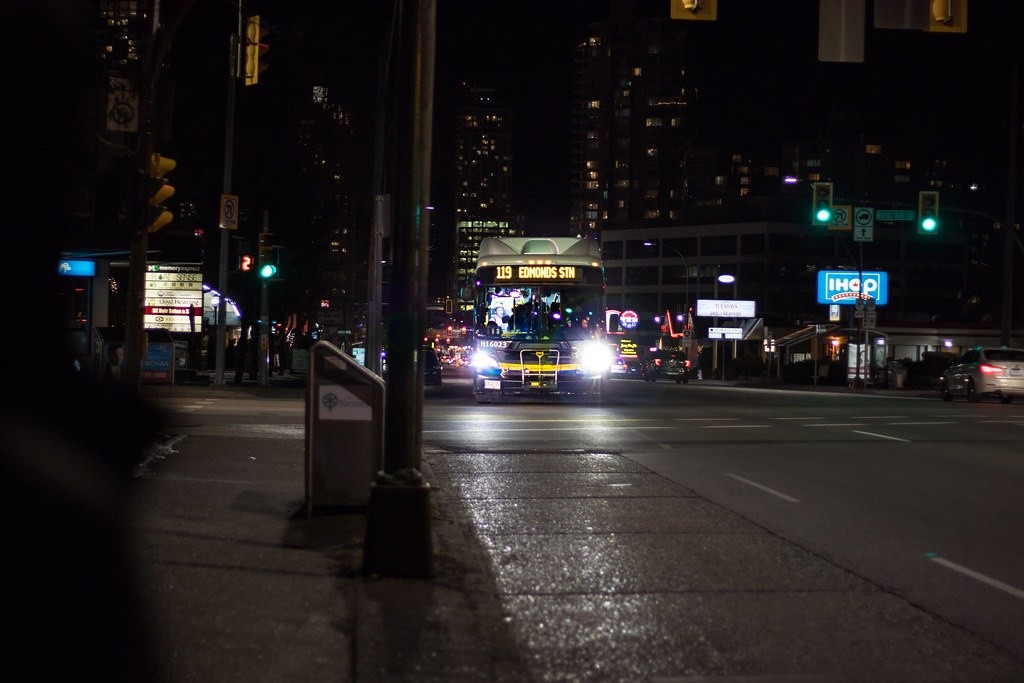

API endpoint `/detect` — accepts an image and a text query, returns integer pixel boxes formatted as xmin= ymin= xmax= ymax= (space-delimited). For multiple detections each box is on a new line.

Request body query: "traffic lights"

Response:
xmin=245 ymin=15 xmax=275 ymax=86
xmin=143 ymin=151 xmax=180 ymax=235
xmin=811 ymin=181 xmax=833 ymax=226
xmin=447 ymin=326 xmax=454 ymax=337
xmin=445 ymin=299 xmax=453 ymax=314
xmin=462 ymin=326 xmax=467 ymax=334
xmin=258 ymin=233 xmax=280 ymax=280
xmin=239 ymin=255 xmax=257 ymax=272
xmin=918 ymin=190 xmax=941 ymax=242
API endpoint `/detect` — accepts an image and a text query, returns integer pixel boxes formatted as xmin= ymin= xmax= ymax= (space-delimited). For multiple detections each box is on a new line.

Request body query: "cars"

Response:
xmin=938 ymin=347 xmax=1024 ymax=404
xmin=422 ymin=348 xmax=445 ymax=385
xmin=643 ymin=350 xmax=693 ymax=383
xmin=440 ymin=353 xmax=472 ymax=365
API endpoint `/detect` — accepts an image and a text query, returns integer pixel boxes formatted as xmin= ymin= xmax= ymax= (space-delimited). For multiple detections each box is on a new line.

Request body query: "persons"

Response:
xmin=106 ymin=342 xmax=176 ymax=444
xmin=509 ymin=305 xmax=527 ymax=332
xmin=491 ymin=306 xmax=504 ymax=326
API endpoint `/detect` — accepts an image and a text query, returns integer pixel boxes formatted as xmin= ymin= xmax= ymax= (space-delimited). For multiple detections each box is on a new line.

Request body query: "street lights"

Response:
xmin=644 ymin=241 xmax=690 ymax=360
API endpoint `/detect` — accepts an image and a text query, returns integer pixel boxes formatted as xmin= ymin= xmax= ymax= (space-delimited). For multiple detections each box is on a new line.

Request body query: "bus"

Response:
xmin=473 ymin=239 xmax=613 ymax=405
